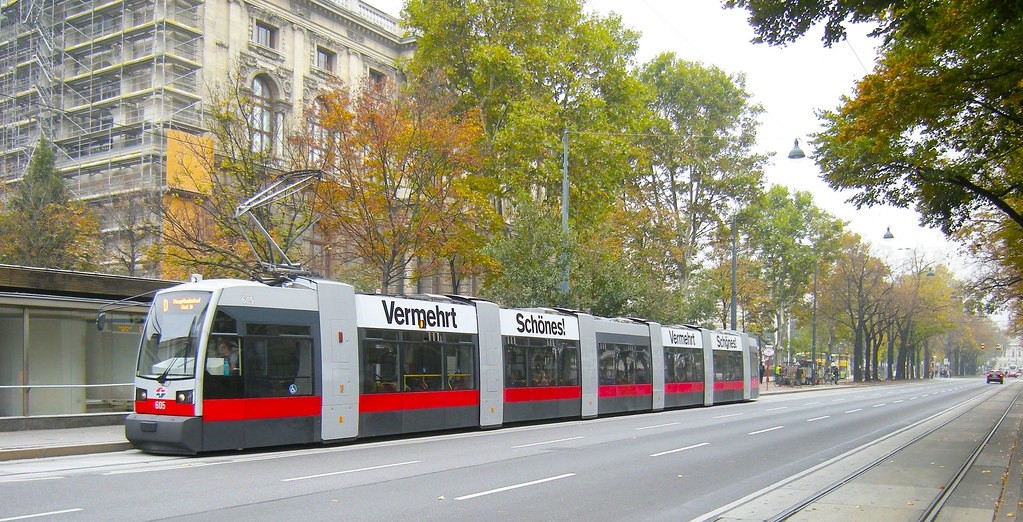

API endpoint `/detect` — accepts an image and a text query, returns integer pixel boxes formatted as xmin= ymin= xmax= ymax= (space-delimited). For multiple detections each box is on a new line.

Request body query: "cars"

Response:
xmin=986 ymin=369 xmax=1004 ymax=384
xmin=1002 ymin=364 xmax=1023 ymax=378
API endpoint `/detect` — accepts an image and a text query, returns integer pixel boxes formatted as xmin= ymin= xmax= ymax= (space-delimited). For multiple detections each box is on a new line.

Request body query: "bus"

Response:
xmin=94 ymin=169 xmax=760 ymax=457
xmin=793 ymin=350 xmax=849 ymax=380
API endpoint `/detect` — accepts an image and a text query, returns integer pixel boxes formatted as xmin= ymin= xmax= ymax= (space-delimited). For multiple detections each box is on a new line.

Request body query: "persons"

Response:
xmin=757 ymin=362 xmax=838 ymax=385
xmin=447 ymin=366 xmax=619 ymax=390
xmin=928 ymin=366 xmax=952 ymax=378
xmin=219 ymin=344 xmax=240 ymax=373
